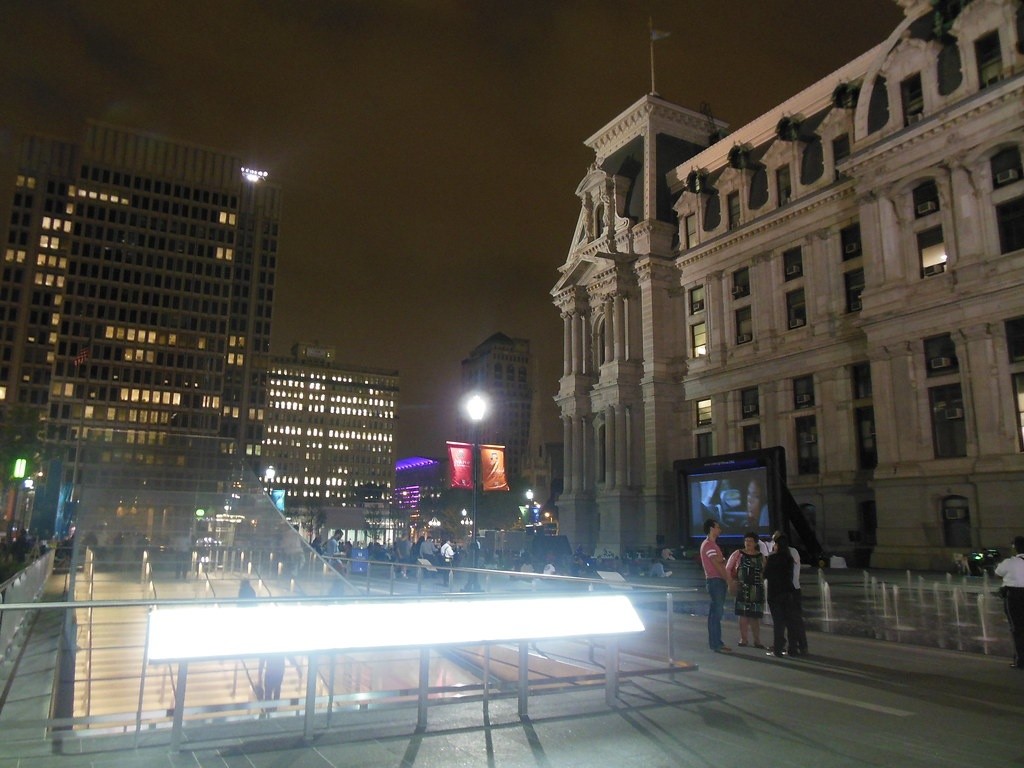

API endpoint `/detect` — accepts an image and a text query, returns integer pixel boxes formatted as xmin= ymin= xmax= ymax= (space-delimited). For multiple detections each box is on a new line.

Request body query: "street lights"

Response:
xmin=4 ymin=458 xmax=27 ymax=562
xmin=253 ymin=465 xmax=279 ymax=575
xmin=454 ymin=386 xmax=495 ymax=594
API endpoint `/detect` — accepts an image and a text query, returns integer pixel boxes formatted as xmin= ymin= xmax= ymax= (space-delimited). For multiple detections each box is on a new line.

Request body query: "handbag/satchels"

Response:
xmin=443 ymin=556 xmax=453 ymax=564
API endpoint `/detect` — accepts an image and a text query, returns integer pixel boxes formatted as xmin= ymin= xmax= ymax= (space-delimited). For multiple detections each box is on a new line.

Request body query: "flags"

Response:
xmin=480 ymin=449 xmax=510 ymax=491
xmin=448 ymin=447 xmax=474 ymax=491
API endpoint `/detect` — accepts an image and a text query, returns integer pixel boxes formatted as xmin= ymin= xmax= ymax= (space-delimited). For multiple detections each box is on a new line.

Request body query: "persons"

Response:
xmin=739 ymin=472 xmax=765 ymax=529
xmin=237 ymin=581 xmax=256 ymax=608
xmin=700 ymin=519 xmax=808 ymax=657
xmin=275 ymin=525 xmax=687 ymax=587
xmin=64 ymin=530 xmax=191 ymax=580
xmin=255 ymin=656 xmax=285 ymax=711
xmin=995 ymin=536 xmax=1024 ymax=668
xmin=0 ymin=530 xmax=46 ymax=575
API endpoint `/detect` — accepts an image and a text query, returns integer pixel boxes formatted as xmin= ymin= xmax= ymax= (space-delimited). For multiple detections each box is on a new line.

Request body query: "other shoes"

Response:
xmin=752 ymin=642 xmax=766 ymax=649
xmin=714 ymin=644 xmax=733 ymax=653
xmin=401 ymin=570 xmax=406 ymax=578
xmin=737 ymin=639 xmax=748 ymax=646
xmin=766 ymin=649 xmax=781 ymax=657
xmin=1010 ymin=663 xmax=1024 ymax=668
